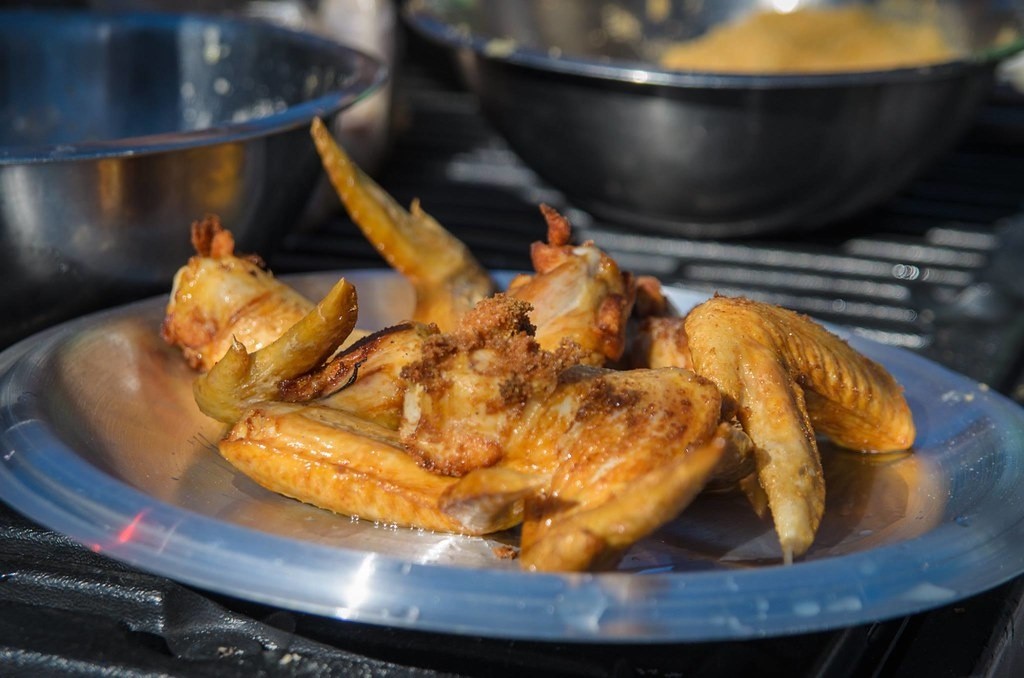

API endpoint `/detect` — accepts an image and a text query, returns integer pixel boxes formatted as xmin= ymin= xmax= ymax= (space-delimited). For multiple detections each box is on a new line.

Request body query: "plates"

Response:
xmin=3 ymin=267 xmax=1024 ymax=648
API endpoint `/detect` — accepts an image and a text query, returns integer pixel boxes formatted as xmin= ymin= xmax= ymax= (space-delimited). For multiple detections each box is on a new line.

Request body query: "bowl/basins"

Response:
xmin=395 ymin=4 xmax=1016 ymax=237
xmin=1 ymin=14 xmax=391 ymax=312
